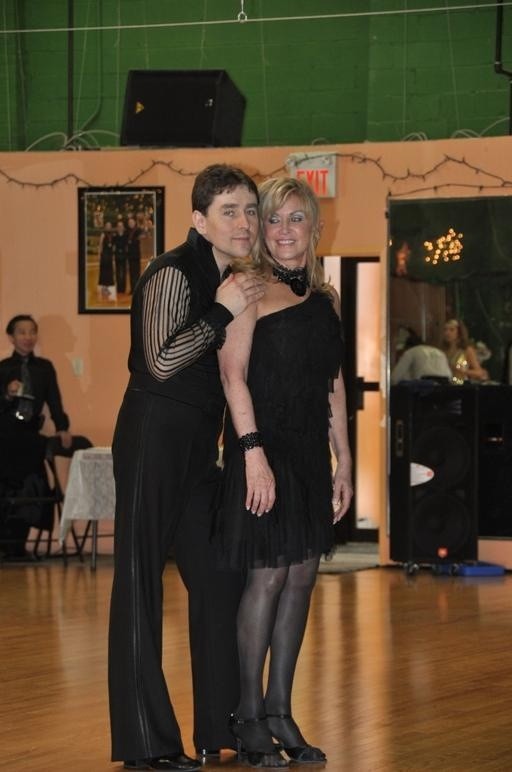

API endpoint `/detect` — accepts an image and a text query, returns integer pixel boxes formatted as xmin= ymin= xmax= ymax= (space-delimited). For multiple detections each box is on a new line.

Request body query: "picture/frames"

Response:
xmin=77 ymin=186 xmax=164 ymax=313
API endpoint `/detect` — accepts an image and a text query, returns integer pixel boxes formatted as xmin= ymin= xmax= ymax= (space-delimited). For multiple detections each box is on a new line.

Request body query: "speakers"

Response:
xmin=390 ymin=386 xmax=478 ymax=563
xmin=478 ymin=386 xmax=512 ymax=538
xmin=121 ymin=69 xmax=246 ymax=146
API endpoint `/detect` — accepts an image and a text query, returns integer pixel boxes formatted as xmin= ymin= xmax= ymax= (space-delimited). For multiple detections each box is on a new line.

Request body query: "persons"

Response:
xmin=1 ymin=313 xmax=73 ymax=564
xmin=215 ymin=175 xmax=355 ymax=769
xmin=439 ymin=315 xmax=489 ymax=385
xmin=85 ymin=194 xmax=153 ymax=303
xmin=391 ymin=334 xmax=451 ymax=388
xmin=107 ymin=162 xmax=268 ymax=772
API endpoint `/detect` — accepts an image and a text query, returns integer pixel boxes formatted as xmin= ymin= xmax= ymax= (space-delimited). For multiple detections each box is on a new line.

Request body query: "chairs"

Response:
xmin=33 ymin=435 xmax=94 ymax=567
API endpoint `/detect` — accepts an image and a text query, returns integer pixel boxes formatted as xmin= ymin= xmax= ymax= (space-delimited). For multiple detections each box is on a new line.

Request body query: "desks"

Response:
xmin=74 ymin=446 xmax=118 ymax=572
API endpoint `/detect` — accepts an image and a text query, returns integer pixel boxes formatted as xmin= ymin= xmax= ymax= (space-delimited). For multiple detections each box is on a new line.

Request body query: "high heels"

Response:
xmin=124 ymin=753 xmax=201 ymax=772
xmin=267 ymin=712 xmax=327 ymax=763
xmin=228 ymin=712 xmax=289 ymax=769
xmin=195 ymin=740 xmax=236 ymax=755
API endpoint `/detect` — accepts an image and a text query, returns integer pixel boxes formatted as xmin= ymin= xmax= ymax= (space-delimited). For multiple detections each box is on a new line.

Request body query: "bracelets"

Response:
xmin=267 ymin=256 xmax=311 ymax=298
xmin=236 ymin=431 xmax=264 ymax=453
